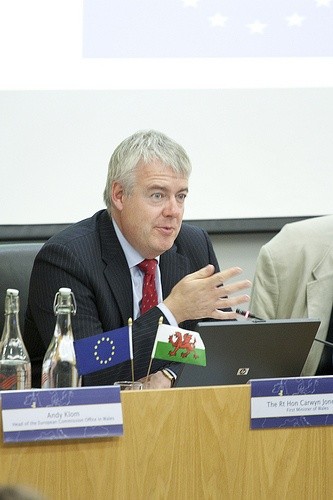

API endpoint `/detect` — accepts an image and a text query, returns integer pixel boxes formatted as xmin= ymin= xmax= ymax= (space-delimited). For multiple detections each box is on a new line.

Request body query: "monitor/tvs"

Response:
xmin=173 ymin=320 xmax=321 ymax=388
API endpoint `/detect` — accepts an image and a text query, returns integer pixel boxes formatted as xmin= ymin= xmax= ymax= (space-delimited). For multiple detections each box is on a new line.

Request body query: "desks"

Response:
xmin=0 ymin=385 xmax=333 ymax=500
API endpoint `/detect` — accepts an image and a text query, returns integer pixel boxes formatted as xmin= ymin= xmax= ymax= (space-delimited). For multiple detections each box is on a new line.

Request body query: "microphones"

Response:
xmin=235 ymin=308 xmax=333 ymax=347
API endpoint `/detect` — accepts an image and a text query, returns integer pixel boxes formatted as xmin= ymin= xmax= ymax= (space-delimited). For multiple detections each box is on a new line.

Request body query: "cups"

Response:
xmin=113 ymin=381 xmax=143 ymax=392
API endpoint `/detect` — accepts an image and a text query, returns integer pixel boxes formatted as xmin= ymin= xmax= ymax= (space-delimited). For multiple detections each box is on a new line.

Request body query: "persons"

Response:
xmin=22 ymin=130 xmax=252 ymax=392
xmin=250 ymin=215 xmax=333 ymax=377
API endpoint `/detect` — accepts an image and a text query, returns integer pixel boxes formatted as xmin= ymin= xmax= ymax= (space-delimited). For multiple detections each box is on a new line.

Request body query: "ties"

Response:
xmin=138 ymin=257 xmax=158 ymax=315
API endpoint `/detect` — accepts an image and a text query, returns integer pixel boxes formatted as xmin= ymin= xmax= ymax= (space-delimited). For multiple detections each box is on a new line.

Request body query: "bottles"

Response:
xmin=0 ymin=288 xmax=31 ymax=390
xmin=42 ymin=287 xmax=82 ymax=388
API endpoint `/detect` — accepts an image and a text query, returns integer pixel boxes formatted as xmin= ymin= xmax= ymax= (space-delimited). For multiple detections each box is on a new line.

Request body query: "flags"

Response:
xmin=150 ymin=324 xmax=207 ymax=367
xmin=74 ymin=325 xmax=133 ymax=377
